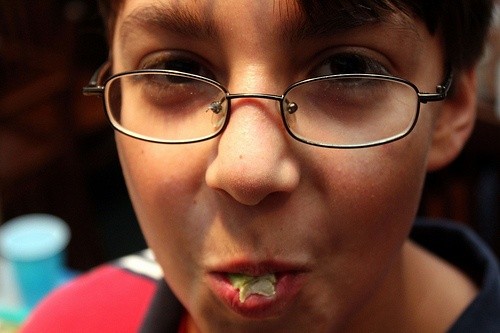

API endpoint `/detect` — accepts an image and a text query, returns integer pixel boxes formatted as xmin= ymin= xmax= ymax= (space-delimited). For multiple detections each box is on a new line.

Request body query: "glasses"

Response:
xmin=82 ymin=58 xmax=455 ymax=149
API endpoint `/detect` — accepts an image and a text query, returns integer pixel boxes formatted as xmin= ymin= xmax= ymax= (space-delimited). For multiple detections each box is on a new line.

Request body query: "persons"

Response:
xmin=18 ymin=0 xmax=500 ymax=333
